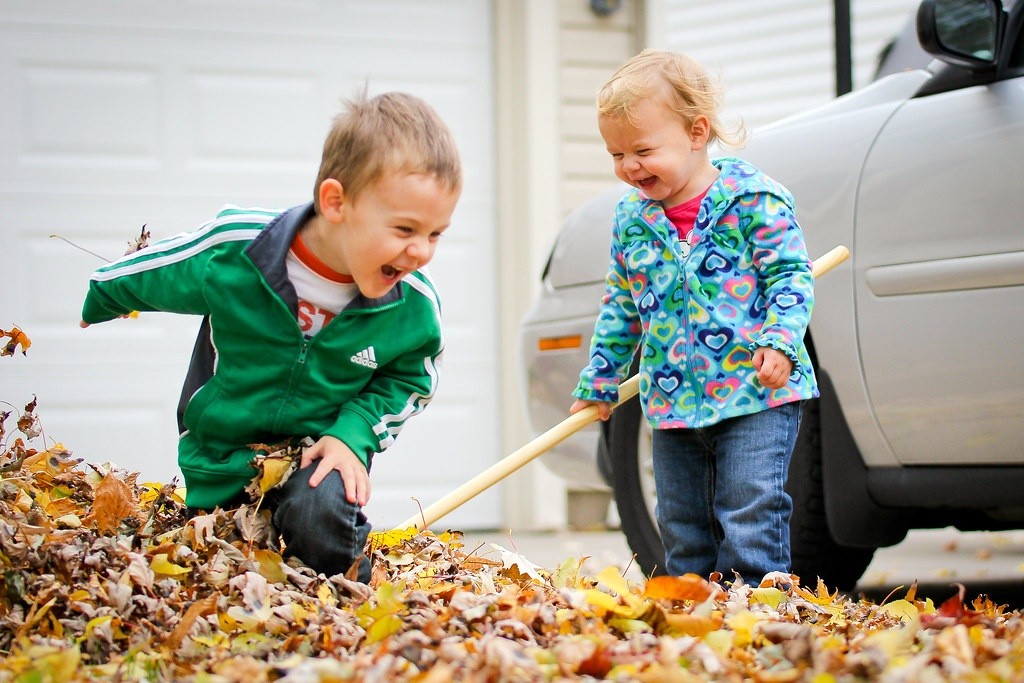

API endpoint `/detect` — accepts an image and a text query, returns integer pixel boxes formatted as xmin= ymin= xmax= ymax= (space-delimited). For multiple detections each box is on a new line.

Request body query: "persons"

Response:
xmin=567 ymin=48 xmax=821 ymax=592
xmin=79 ymin=77 xmax=464 ymax=583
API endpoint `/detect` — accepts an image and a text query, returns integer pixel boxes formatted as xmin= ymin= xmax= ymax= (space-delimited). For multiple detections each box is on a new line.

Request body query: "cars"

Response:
xmin=522 ymin=0 xmax=1024 ymax=589
